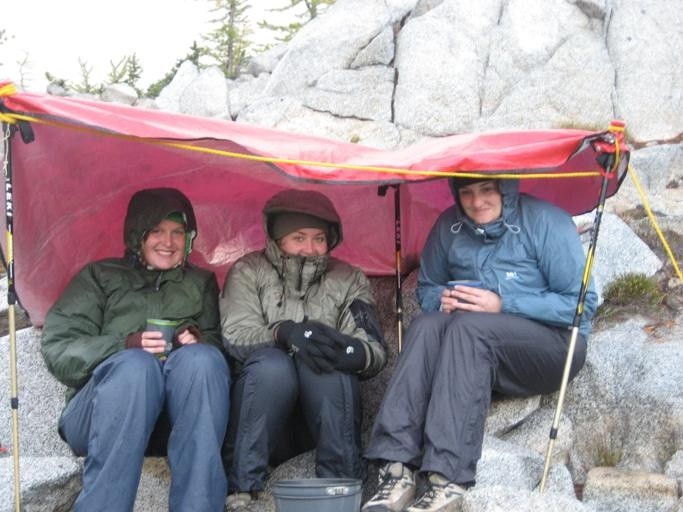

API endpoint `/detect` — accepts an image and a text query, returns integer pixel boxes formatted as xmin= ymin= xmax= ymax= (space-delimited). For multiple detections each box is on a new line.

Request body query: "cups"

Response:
xmin=446 ymin=280 xmax=482 ymax=311
xmin=145 ymin=318 xmax=177 ymax=362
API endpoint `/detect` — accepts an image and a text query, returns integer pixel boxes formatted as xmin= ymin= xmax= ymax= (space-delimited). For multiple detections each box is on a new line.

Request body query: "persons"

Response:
xmin=361 ymin=175 xmax=598 ymax=511
xmin=219 ymin=188 xmax=388 ymax=512
xmin=41 ymin=187 xmax=228 ymax=512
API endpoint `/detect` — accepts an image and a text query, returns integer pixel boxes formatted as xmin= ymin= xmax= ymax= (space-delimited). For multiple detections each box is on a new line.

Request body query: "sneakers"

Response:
xmin=406 ymin=473 xmax=470 ymax=512
xmin=361 ymin=462 xmax=415 ymax=512
xmin=225 ymin=493 xmax=251 ymax=512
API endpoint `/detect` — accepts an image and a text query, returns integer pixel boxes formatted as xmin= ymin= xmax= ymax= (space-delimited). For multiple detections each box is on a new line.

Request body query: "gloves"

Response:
xmin=313 ymin=321 xmax=366 ymax=373
xmin=277 ymin=321 xmax=335 ymax=374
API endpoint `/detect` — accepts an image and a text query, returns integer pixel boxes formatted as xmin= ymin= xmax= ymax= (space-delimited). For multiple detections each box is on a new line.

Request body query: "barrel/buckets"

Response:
xmin=265 ymin=477 xmax=362 ymax=511
